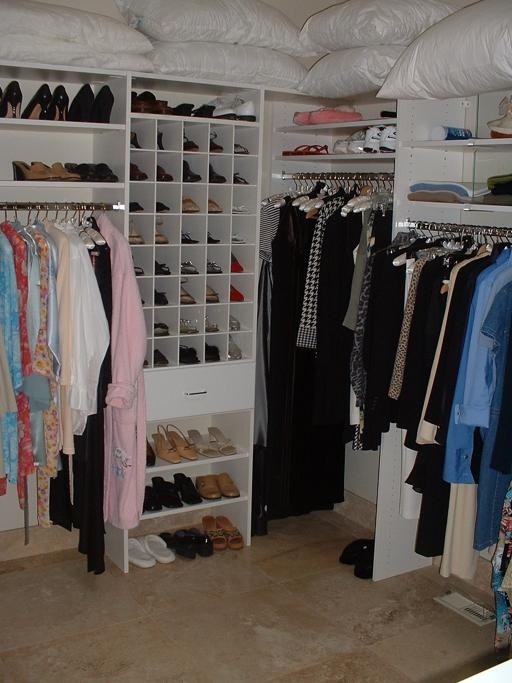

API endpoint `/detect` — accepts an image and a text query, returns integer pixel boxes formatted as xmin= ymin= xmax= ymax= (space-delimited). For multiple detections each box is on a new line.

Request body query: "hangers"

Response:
xmin=2 ymin=202 xmax=127 ymax=248
xmin=375 ymin=220 xmax=512 ymax=291
xmin=262 ymin=173 xmax=394 ymax=216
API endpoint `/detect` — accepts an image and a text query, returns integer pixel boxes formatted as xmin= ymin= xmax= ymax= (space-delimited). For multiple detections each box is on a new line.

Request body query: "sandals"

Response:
xmin=147 ymin=424 xmax=237 ymax=466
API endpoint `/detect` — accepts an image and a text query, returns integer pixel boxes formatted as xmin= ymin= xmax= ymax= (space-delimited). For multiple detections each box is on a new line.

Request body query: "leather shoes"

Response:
xmin=142 ymin=473 xmax=240 ymax=511
xmin=131 ymin=91 xmax=256 ymax=121
xmin=129 ymin=133 xmax=248 ymax=365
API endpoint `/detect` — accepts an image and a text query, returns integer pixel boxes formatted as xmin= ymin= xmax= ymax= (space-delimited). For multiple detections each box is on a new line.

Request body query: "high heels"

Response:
xmin=0 ymin=81 xmax=114 ymax=123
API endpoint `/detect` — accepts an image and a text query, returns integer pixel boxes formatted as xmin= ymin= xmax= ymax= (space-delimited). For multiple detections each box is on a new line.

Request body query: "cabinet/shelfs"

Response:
xmin=0 ymin=60 xmax=512 ymax=582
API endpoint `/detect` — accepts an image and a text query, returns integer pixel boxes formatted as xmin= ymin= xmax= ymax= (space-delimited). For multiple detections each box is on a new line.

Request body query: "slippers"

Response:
xmin=283 ymin=145 xmax=328 ymax=155
xmin=129 ymin=516 xmax=243 ymax=568
xmin=13 ymin=161 xmax=118 ymax=182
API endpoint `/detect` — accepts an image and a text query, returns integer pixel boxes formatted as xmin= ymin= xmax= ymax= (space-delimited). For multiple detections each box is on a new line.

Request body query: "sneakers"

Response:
xmin=334 ymin=126 xmax=396 ymax=153
xmin=293 ymin=111 xmax=362 ymax=124
